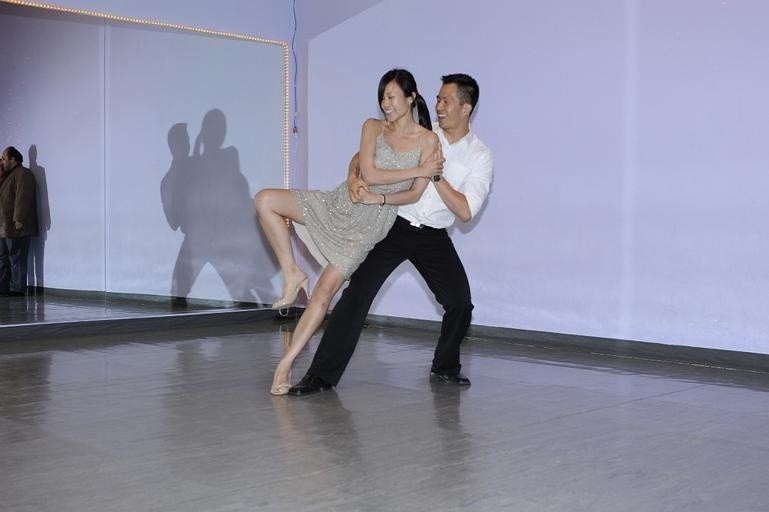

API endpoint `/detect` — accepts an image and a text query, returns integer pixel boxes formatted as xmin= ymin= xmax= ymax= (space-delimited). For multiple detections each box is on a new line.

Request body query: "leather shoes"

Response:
xmin=430 ymin=371 xmax=470 ymax=385
xmin=289 ymin=374 xmax=332 ymax=396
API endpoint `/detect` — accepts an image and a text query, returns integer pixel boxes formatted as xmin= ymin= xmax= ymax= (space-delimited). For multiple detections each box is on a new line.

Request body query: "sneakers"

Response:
xmin=0 ymin=291 xmax=25 ymax=297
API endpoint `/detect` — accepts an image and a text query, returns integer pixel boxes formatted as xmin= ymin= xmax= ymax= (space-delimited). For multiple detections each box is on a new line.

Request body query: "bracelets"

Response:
xmin=379 ymin=193 xmax=386 ymax=207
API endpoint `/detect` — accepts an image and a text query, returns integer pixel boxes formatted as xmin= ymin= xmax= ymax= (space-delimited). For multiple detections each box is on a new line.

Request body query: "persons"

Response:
xmin=253 ymin=68 xmax=446 ymax=396
xmin=288 ymin=73 xmax=493 ymax=398
xmin=0 ymin=146 xmax=40 ymax=297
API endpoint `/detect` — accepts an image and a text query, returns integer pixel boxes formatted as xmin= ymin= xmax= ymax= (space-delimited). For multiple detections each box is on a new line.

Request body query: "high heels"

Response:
xmin=272 ymin=277 xmax=309 ymax=311
xmin=271 ymin=366 xmax=292 ymax=395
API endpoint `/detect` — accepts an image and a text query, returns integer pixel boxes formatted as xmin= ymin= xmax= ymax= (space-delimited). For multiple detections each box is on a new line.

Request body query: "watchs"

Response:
xmin=430 ymin=174 xmax=442 ymax=183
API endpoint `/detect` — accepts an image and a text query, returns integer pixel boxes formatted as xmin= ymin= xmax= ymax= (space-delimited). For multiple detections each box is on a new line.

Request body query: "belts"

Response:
xmin=409 ymin=220 xmax=423 ymax=229
